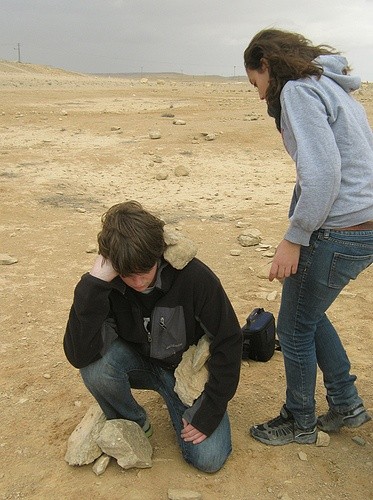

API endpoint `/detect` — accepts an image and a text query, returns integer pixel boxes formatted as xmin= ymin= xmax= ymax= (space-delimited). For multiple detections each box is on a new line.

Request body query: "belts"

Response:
xmin=335 ymin=222 xmax=373 ymax=230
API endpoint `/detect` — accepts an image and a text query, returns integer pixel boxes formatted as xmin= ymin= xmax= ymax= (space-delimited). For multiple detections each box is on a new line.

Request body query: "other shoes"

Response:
xmin=144 ymin=424 xmax=152 ymax=438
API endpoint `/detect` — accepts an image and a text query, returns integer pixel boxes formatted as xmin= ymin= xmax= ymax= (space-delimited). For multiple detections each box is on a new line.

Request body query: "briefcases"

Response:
xmin=242 ymin=308 xmax=276 ymax=362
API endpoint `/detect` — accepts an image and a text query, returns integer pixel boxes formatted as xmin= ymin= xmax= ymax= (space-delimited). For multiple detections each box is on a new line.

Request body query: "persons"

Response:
xmin=241 ymin=28 xmax=372 ymax=446
xmin=61 ymin=200 xmax=243 ymax=474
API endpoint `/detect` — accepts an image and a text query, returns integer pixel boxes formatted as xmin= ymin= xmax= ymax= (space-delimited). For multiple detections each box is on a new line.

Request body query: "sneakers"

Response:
xmin=317 ymin=396 xmax=371 ymax=431
xmin=250 ymin=404 xmax=317 ymax=446
